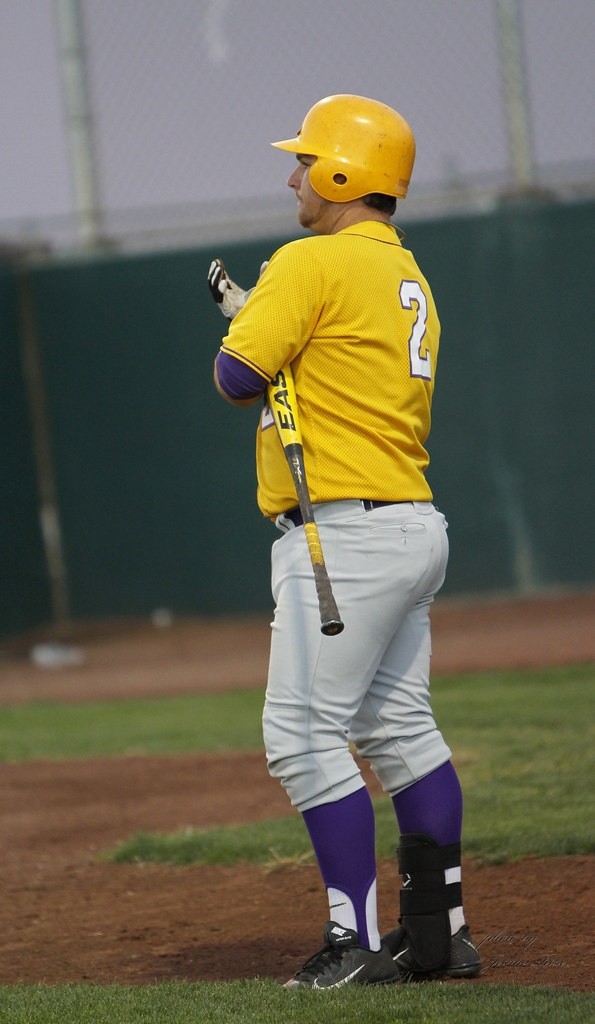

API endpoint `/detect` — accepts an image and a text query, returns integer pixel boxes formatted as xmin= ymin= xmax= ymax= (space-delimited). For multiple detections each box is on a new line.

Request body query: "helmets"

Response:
xmin=271 ymin=93 xmax=416 ymax=203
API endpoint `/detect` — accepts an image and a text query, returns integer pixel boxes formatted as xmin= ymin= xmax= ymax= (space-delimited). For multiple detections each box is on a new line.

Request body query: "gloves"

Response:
xmin=209 ymin=259 xmax=270 ymax=321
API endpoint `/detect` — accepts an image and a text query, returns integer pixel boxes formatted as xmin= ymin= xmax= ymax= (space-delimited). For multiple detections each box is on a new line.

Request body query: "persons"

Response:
xmin=206 ymin=93 xmax=484 ymax=989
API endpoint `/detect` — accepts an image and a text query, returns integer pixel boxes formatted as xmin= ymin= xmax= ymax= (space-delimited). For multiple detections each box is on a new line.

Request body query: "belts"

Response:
xmin=277 ymin=499 xmax=412 ymax=532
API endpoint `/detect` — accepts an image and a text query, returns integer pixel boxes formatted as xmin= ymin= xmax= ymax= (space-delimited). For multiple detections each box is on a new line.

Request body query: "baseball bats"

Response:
xmin=244 ymin=284 xmax=347 ymax=639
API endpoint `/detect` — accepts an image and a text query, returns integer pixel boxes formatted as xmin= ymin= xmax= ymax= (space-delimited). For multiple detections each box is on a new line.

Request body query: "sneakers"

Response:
xmin=393 ymin=925 xmax=482 ymax=978
xmin=283 ymin=921 xmax=402 ymax=990
